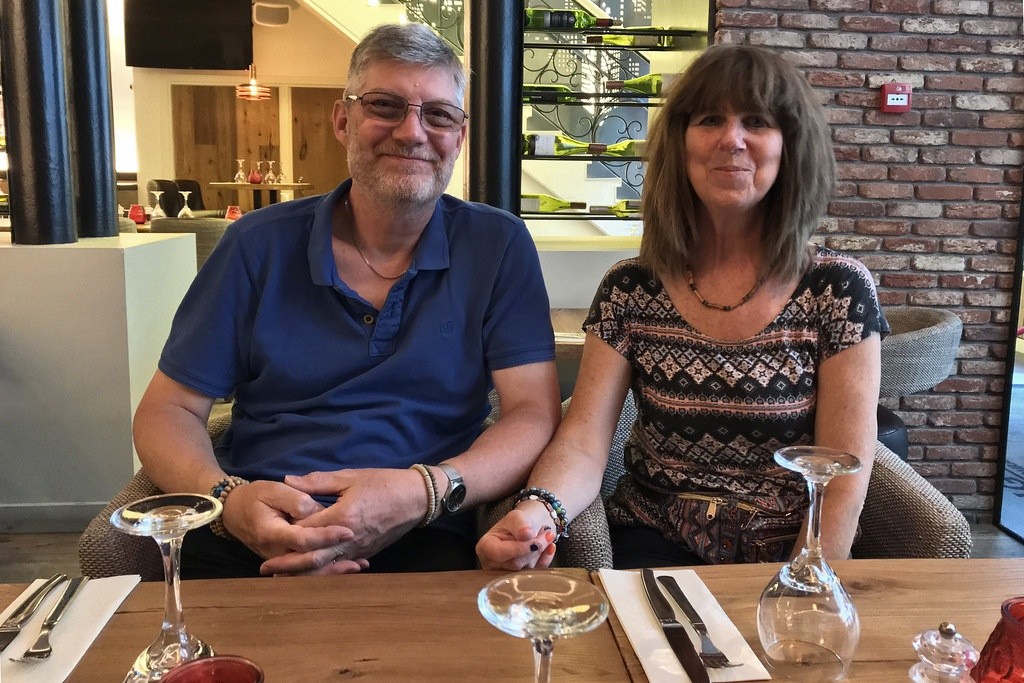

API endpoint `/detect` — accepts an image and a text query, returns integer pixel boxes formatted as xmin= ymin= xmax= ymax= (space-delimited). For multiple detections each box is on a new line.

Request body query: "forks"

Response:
xmin=657 ymin=576 xmax=743 ymax=669
xmin=10 ymin=574 xmax=91 ymax=661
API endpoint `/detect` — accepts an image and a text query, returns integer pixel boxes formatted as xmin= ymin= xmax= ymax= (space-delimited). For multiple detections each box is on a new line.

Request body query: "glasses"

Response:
xmin=346 ymin=92 xmax=469 ymax=131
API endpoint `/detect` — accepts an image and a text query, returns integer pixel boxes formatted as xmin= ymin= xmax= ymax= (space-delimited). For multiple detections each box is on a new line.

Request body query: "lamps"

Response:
xmin=235 ymin=63 xmax=272 ymax=101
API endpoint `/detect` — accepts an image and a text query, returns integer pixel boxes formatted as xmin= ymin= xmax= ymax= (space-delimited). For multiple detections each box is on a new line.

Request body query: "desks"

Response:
xmin=208 ymin=182 xmax=315 ymax=207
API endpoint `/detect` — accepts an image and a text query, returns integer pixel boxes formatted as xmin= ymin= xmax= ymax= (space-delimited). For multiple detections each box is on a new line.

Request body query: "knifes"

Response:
xmin=641 ymin=569 xmax=710 ymax=683
xmin=0 ymin=573 xmax=68 ymax=652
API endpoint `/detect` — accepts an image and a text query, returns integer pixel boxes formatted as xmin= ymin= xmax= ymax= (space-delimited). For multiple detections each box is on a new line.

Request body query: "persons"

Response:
xmin=132 ymin=23 xmax=562 ymax=578
xmin=475 ymin=42 xmax=892 ymax=570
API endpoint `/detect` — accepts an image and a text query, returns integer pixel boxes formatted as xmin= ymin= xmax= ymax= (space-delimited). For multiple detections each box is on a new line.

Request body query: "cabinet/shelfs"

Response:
xmin=517 ymin=0 xmax=718 ymax=221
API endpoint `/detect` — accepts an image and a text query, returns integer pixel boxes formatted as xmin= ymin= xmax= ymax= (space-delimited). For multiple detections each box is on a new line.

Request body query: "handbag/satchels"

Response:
xmin=628 ymin=473 xmax=861 ymax=565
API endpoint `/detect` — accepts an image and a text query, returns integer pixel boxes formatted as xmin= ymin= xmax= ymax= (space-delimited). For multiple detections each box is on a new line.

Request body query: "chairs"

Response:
xmin=78 ymin=412 xmax=600 ymax=578
xmin=561 ymin=388 xmax=974 ymax=576
xmin=118 ymin=179 xmax=235 ymax=275
xmin=878 ymin=303 xmax=962 ymax=462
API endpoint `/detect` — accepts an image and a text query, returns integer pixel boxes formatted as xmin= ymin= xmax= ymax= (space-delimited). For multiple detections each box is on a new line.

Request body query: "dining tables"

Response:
xmin=0 ymin=557 xmax=1024 ymax=683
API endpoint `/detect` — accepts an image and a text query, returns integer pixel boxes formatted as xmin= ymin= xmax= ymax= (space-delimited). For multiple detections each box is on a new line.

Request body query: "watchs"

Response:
xmin=436 ymin=464 xmax=466 ymax=512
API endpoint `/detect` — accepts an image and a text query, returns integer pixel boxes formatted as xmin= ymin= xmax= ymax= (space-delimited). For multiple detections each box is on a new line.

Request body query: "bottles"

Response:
xmin=589 ymin=140 xmax=648 ymax=156
xmin=521 ymin=195 xmax=586 ymax=212
xmin=908 ymin=623 xmax=980 ymax=683
xmin=606 ymin=72 xmax=682 ymax=91
xmin=590 ymin=198 xmax=643 ymax=217
xmin=586 ymin=24 xmax=665 ymax=45
xmin=524 ymin=8 xmax=622 ymax=32
xmin=970 ymin=596 xmax=1024 ymax=683
xmin=522 ymin=85 xmax=589 ymax=103
xmin=522 ymin=134 xmax=604 ymax=155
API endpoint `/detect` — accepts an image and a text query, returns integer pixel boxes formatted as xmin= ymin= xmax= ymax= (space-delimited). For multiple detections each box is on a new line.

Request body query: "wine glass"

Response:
xmin=477 ymin=572 xmax=609 ymax=683
xmin=757 ymin=446 xmax=861 ymax=683
xmin=177 ymin=191 xmax=193 ymax=219
xmin=110 ymin=492 xmax=223 ymax=683
xmin=150 ymin=191 xmax=166 ymax=221
xmin=234 ymin=158 xmax=288 ymax=184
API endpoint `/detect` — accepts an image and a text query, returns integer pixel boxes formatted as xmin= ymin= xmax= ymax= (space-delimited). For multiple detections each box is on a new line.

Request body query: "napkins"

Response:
xmin=0 ymin=575 xmax=141 ymax=683
xmin=598 ymin=569 xmax=772 ymax=683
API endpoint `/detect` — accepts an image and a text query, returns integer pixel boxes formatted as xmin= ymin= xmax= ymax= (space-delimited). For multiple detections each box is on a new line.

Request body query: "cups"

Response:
xmin=128 ymin=204 xmax=147 ymax=224
xmin=225 ymin=205 xmax=241 ymax=221
xmin=159 ymin=655 xmax=264 ymax=683
xmin=249 ymin=169 xmax=263 ymax=184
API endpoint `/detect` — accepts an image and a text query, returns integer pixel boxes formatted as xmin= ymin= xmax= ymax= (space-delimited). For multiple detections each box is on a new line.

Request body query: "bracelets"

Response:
xmin=514 ymin=487 xmax=570 ymax=543
xmin=208 ymin=476 xmax=249 ymax=540
xmin=410 ymin=464 xmax=439 ymax=527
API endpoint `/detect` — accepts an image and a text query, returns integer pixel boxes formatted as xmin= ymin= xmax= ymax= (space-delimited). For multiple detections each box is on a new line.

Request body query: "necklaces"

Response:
xmin=345 ymin=199 xmax=409 ymax=279
xmin=686 ymin=264 xmax=773 ymax=311
